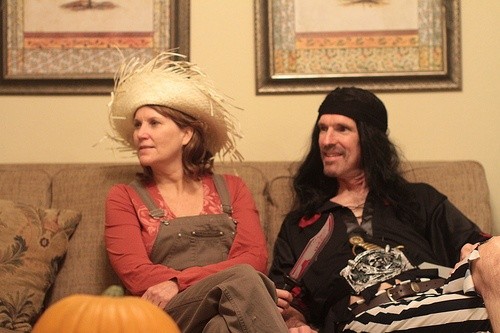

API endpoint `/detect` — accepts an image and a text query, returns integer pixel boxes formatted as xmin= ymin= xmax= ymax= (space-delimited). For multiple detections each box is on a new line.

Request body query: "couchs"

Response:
xmin=0 ymin=161 xmax=496 ymax=333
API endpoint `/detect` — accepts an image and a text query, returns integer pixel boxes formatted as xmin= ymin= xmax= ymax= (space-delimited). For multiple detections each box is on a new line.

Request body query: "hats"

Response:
xmin=318 ymin=87 xmax=388 ymax=131
xmin=108 ymin=48 xmax=244 ymax=163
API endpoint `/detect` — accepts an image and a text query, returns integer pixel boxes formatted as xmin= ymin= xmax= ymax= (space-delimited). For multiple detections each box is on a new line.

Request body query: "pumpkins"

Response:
xmin=30 ymin=285 xmax=182 ymax=333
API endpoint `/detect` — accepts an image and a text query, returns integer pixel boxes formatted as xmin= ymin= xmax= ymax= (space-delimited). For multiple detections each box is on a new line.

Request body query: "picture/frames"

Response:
xmin=254 ymin=0 xmax=465 ymax=96
xmin=0 ymin=0 xmax=191 ymax=97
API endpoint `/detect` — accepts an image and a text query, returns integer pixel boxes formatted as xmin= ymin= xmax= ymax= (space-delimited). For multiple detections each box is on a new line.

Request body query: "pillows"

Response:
xmin=0 ymin=200 xmax=82 ymax=332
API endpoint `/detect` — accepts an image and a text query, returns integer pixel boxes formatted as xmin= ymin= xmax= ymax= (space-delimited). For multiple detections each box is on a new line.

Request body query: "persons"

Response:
xmin=268 ymin=86 xmax=500 ymax=333
xmin=104 ymin=95 xmax=294 ymax=333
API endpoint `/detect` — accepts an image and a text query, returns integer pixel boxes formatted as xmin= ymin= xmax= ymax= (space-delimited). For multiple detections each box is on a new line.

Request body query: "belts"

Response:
xmin=343 ymin=278 xmax=444 ymax=323
